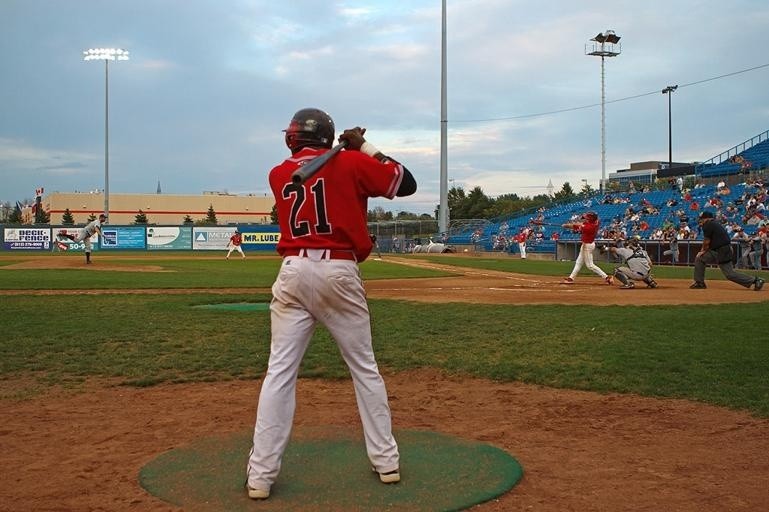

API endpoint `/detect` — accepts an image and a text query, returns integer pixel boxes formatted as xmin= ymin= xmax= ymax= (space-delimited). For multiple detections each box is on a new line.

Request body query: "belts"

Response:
xmin=281 ymin=247 xmax=359 ymax=263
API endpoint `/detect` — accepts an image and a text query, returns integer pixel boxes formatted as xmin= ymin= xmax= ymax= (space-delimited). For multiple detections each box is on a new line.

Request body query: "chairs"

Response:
xmin=439 ymin=209 xmax=550 ymax=252
xmin=550 ymin=179 xmax=769 ymax=252
xmin=704 ymin=138 xmax=769 ymax=177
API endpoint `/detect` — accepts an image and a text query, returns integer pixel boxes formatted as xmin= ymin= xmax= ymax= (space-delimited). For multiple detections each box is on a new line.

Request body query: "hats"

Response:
xmin=98 ymin=213 xmax=108 ymax=219
xmin=696 ymin=210 xmax=716 ymax=219
xmin=737 ymin=227 xmax=757 ymax=235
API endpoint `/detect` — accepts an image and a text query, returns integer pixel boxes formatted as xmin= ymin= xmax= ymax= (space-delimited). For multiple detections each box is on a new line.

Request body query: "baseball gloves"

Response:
xmin=599 ymin=246 xmax=609 ymax=256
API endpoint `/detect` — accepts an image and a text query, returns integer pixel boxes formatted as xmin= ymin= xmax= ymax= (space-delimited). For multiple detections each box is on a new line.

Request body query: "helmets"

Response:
xmin=582 ymin=212 xmax=598 ymax=220
xmin=623 ymin=237 xmax=639 ymax=249
xmin=280 ymin=107 xmax=335 ymax=155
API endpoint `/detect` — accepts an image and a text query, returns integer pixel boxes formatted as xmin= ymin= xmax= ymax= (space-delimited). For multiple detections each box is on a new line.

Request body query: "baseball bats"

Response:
xmin=291 ymin=129 xmax=366 ymax=186
xmin=531 ymin=220 xmax=561 ymax=227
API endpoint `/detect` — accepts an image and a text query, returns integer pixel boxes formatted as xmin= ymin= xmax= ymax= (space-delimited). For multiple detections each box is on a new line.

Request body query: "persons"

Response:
xmin=225 ymin=230 xmax=246 ymax=261
xmin=369 ymin=232 xmax=381 ymax=258
xmin=57 ymin=214 xmax=108 ymax=264
xmin=244 ymin=109 xmax=417 ymax=499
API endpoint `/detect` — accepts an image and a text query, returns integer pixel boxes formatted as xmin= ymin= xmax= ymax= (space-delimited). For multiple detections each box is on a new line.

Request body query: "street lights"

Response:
xmin=661 ymin=85 xmax=678 ymax=167
xmin=82 ymin=46 xmax=130 ymax=224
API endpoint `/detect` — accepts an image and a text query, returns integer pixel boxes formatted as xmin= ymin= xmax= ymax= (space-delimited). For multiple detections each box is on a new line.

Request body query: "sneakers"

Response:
xmin=645 ymin=280 xmax=658 ymax=289
xmin=559 ymin=276 xmax=575 ymax=285
xmin=688 ymin=280 xmax=707 ymax=290
xmin=369 ymin=460 xmax=403 ymax=485
xmin=605 ymin=274 xmax=615 ymax=285
xmin=616 ymin=281 xmax=634 ymax=289
xmin=752 ymin=277 xmax=765 ymax=292
xmin=242 ymin=477 xmax=273 ymax=500
